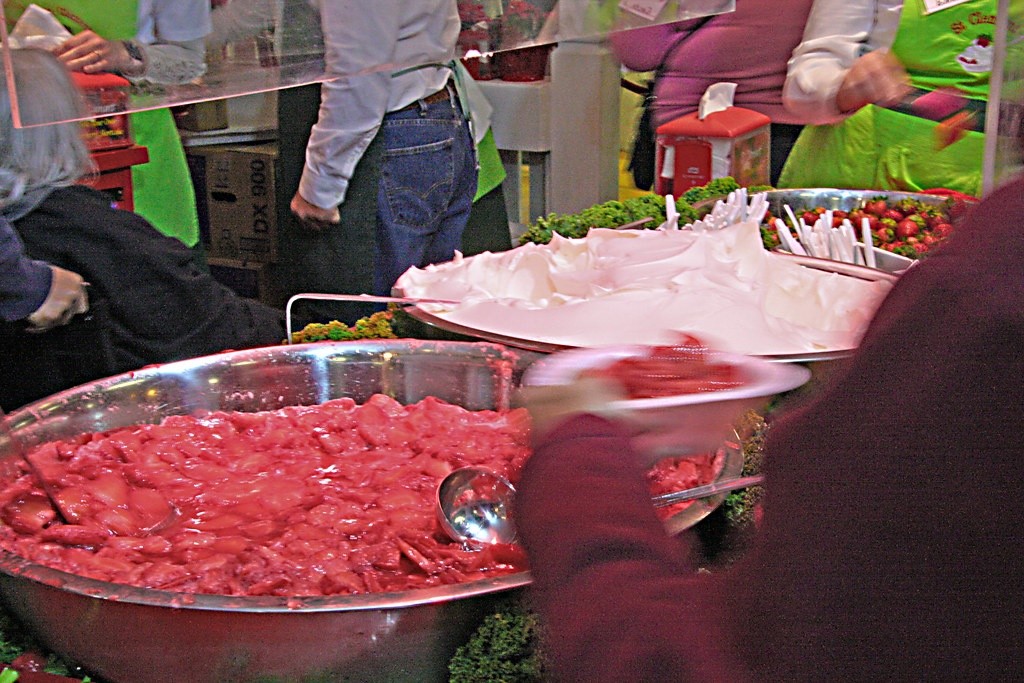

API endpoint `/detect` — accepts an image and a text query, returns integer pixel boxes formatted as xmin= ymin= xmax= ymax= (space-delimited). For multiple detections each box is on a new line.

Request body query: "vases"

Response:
xmin=456 ymin=30 xmax=495 ymax=79
xmin=499 ymin=35 xmax=549 ymax=82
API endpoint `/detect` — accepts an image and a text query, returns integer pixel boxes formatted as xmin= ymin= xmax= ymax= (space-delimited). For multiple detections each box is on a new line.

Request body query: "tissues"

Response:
xmin=0 ymin=4 xmax=134 ymax=154
xmin=654 ymin=82 xmax=772 ymax=203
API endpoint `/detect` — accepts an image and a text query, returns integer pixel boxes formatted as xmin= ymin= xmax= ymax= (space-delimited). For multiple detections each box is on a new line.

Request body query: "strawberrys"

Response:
xmin=758 ymin=195 xmax=961 ymax=259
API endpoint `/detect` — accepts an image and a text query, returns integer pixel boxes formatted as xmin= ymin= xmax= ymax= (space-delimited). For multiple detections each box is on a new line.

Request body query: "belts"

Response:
xmin=399 ymin=80 xmax=458 ymax=112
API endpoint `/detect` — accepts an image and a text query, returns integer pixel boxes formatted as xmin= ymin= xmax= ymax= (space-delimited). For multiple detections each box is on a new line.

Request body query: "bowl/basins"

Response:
xmin=691 ymin=188 xmax=949 ymax=258
xmin=521 ymin=345 xmax=814 ymax=455
xmin=1 ymin=339 xmax=547 ymax=683
xmin=394 ymin=253 xmax=901 ymax=363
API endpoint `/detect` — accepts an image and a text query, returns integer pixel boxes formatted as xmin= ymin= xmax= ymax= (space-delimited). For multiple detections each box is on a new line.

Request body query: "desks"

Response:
xmin=68 ymin=144 xmax=152 ymax=213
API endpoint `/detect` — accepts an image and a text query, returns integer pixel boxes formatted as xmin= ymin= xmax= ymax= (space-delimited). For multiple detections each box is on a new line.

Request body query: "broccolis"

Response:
xmin=518 ymin=175 xmax=783 ymax=250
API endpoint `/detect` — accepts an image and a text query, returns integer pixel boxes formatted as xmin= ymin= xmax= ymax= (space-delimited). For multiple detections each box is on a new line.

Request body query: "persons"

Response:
xmin=1 ymin=0 xmax=1024 ymax=357
xmin=513 ymin=159 xmax=1024 ymax=683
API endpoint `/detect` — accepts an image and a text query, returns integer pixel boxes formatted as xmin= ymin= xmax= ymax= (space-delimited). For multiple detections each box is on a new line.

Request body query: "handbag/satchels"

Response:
xmin=627 ymin=104 xmax=654 ymax=190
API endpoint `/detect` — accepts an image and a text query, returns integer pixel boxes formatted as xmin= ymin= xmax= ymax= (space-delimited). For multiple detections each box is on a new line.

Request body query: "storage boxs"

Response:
xmin=205 ymin=143 xmax=281 ymax=262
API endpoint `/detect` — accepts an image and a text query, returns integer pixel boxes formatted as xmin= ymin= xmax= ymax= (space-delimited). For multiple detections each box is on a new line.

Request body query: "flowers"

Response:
xmin=458 ymin=4 xmax=493 ymax=31
xmin=499 ymin=1 xmax=556 ymax=41
